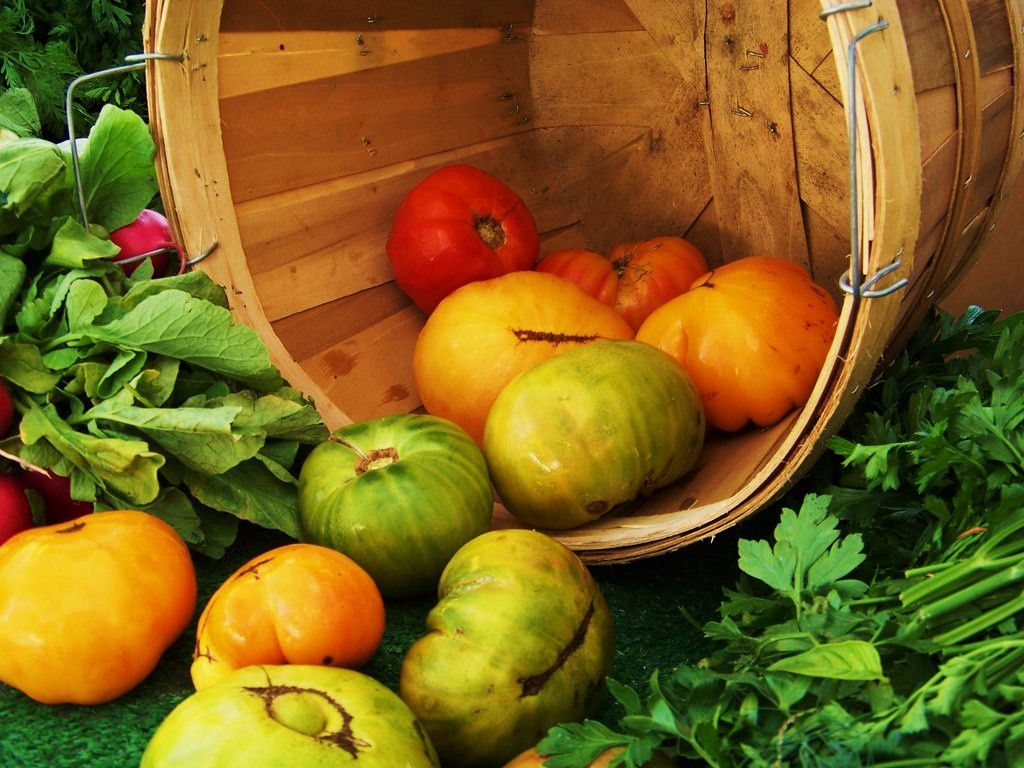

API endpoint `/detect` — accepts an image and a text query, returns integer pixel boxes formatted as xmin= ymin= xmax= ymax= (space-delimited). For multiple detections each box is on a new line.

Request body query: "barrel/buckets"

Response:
xmin=128 ymin=0 xmax=1022 ymax=561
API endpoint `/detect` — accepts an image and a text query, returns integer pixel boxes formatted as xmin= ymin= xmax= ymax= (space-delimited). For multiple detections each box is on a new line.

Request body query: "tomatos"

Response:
xmin=0 ymin=511 xmax=197 ymax=703
xmin=141 ymin=665 xmax=445 ymax=768
xmin=635 ymin=255 xmax=840 ymax=433
xmin=412 ymin=271 xmax=637 ymax=452
xmin=536 ymin=237 xmax=711 ymax=333
xmin=297 ymin=415 xmax=494 ymax=601
xmin=106 ymin=206 xmax=179 ymax=282
xmin=386 ymin=162 xmax=540 ymax=318
xmin=191 ymin=542 xmax=386 ymax=694
xmin=482 ymin=341 xmax=707 ymax=529
xmin=400 ymin=528 xmax=616 ymax=768
xmin=0 ymin=377 xmax=94 ymax=547
